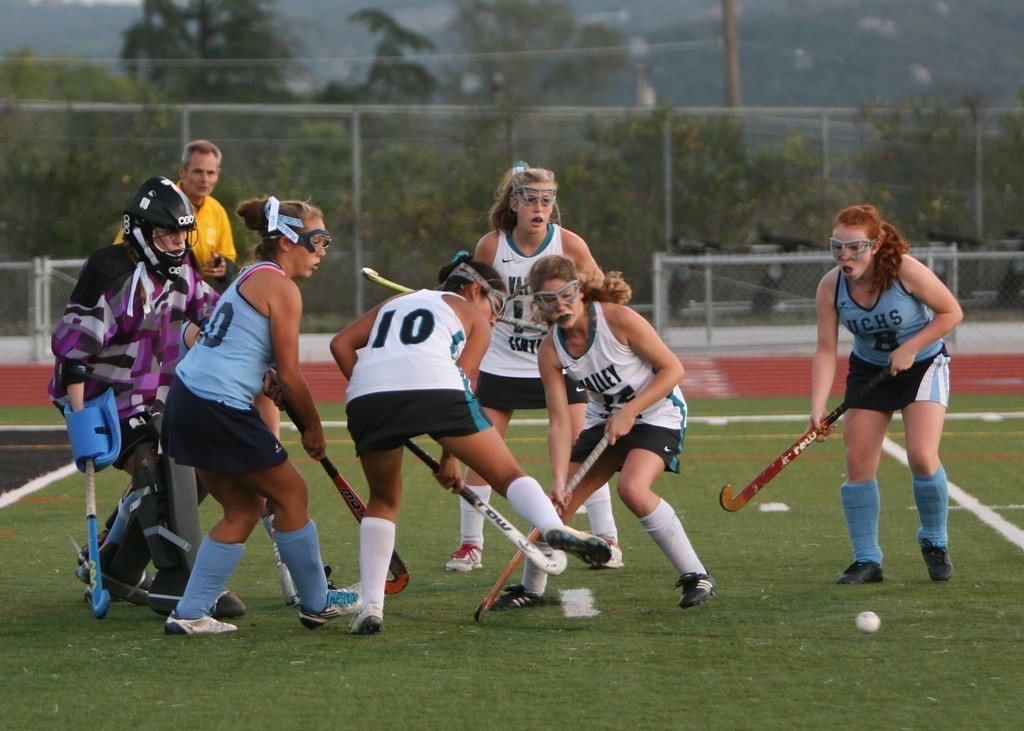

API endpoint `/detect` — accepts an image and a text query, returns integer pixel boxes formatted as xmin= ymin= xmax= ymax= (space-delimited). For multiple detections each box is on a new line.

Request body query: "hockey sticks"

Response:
xmin=404 ymin=439 xmax=569 ymax=577
xmin=85 ymin=459 xmax=111 ymax=618
xmin=268 ymin=382 xmax=410 ymax=596
xmin=360 ymin=266 xmax=552 ymax=333
xmin=719 ymin=361 xmax=894 ymax=513
xmin=471 ymin=429 xmax=611 ymax=623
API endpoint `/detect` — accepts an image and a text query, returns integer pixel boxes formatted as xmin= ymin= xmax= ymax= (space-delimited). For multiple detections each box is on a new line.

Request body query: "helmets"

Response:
xmin=123 ymin=176 xmax=199 ymax=283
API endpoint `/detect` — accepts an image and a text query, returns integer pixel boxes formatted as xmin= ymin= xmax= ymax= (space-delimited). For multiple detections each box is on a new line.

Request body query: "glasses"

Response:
xmin=534 ymin=280 xmax=580 ymax=311
xmin=513 ymin=185 xmax=556 ymax=206
xmin=262 ymin=229 xmax=332 ymax=254
xmin=457 ymin=264 xmax=507 ymax=321
xmin=830 ymin=236 xmax=879 ymax=261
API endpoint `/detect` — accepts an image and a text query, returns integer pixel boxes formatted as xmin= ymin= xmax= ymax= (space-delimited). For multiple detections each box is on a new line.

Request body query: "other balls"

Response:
xmin=855 ymin=609 xmax=881 ymax=633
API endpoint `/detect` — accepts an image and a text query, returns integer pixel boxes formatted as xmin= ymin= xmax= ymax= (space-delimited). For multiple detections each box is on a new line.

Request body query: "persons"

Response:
xmin=442 ymin=164 xmax=632 ymax=573
xmin=804 ymin=205 xmax=964 ymax=584
xmin=161 ymin=197 xmax=364 ymax=635
xmin=46 ymin=138 xmax=334 ymax=618
xmin=491 ymin=256 xmax=717 ymax=611
xmin=328 ymin=252 xmax=612 ymax=635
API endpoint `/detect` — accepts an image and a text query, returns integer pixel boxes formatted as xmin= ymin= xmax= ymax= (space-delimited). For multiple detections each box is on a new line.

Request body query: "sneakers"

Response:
xmin=446 ymin=543 xmax=482 ymax=572
xmin=165 ymin=609 xmax=237 ymax=635
xmin=489 ymin=583 xmax=545 ymax=610
xmin=347 ymin=603 xmax=383 ymax=634
xmin=77 ymin=530 xmax=110 ymax=566
xmin=673 ymin=568 xmax=717 ymax=610
xmin=298 ymin=582 xmax=362 ymax=632
xmin=541 ymin=525 xmax=612 ymax=566
xmin=590 ymin=534 xmax=625 ymax=569
xmin=837 ymin=560 xmax=884 ymax=585
xmin=921 ymin=538 xmax=952 ymax=581
